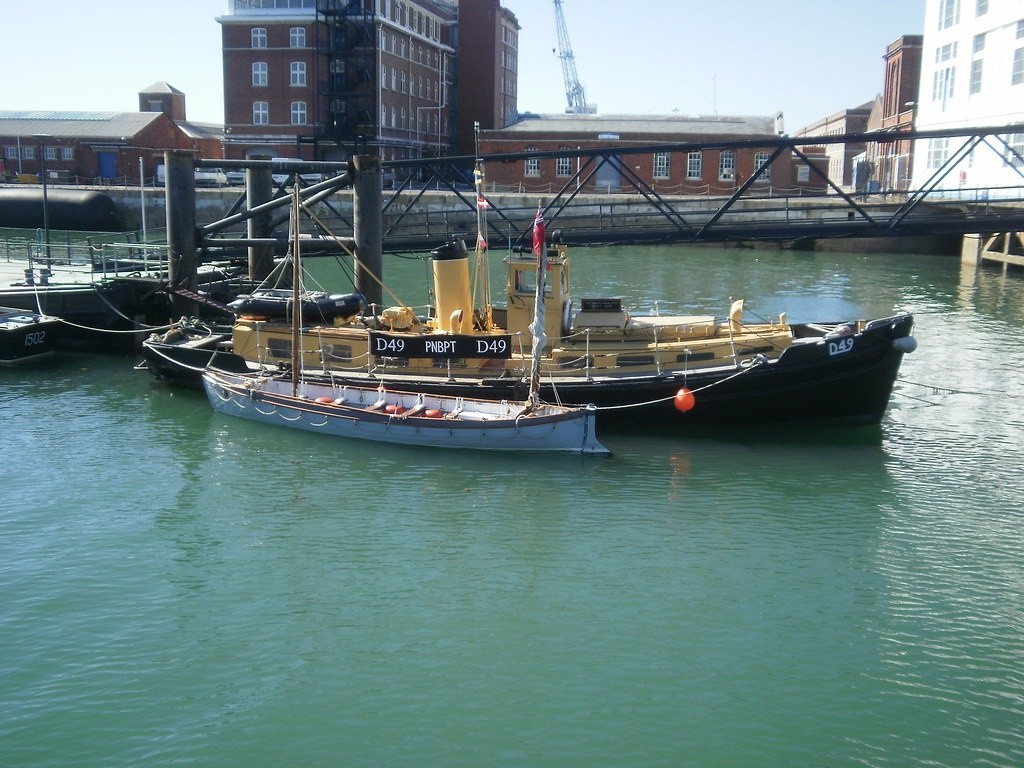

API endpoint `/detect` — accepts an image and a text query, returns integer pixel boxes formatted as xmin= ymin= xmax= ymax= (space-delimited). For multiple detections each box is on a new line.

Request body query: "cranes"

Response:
xmin=543 ymin=0 xmax=598 ymax=116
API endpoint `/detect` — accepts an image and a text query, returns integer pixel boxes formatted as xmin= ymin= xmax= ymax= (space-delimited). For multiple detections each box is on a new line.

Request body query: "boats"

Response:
xmin=141 ymin=119 xmax=916 ymax=442
xmin=198 ymin=119 xmax=614 ymax=466
